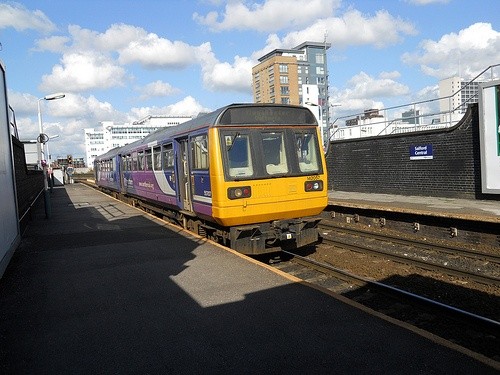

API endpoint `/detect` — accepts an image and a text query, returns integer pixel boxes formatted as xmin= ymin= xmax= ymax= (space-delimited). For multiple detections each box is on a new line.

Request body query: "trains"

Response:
xmin=93 ymin=102 xmax=329 ymax=255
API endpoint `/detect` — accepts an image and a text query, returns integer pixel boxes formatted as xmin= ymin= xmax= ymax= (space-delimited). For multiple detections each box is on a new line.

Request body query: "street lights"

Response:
xmin=37 ymin=90 xmax=66 ymax=228
xmin=45 ymin=134 xmax=60 ymax=193
